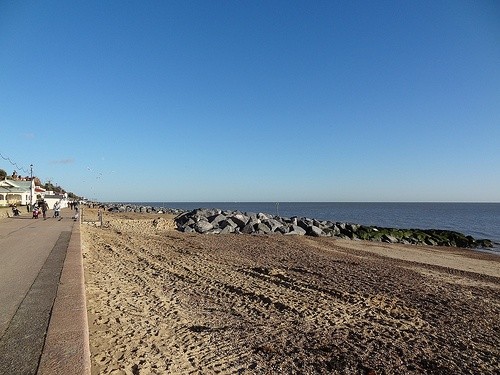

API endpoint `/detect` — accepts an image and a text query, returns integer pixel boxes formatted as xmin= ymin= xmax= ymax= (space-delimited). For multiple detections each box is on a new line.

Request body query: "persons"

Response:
xmin=10 ymin=197 xmax=84 ymax=219
